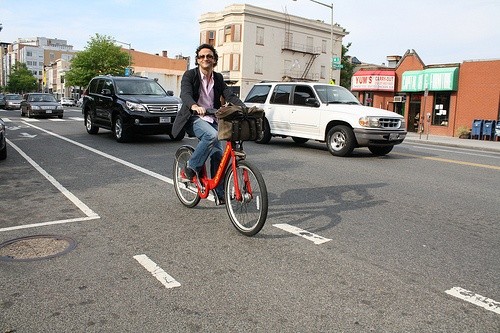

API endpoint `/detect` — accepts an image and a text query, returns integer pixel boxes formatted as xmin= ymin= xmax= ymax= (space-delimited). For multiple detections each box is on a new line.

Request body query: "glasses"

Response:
xmin=197 ymin=55 xmax=214 ymax=59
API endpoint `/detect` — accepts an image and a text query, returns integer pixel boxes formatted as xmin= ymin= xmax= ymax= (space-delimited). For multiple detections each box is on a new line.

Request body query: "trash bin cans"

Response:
xmin=471 ymin=119 xmax=500 ymax=141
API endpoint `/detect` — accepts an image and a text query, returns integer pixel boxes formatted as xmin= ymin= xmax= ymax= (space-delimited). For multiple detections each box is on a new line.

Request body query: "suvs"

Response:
xmin=81 ymin=74 xmax=185 ymax=143
xmin=243 ymin=80 xmax=408 ymax=157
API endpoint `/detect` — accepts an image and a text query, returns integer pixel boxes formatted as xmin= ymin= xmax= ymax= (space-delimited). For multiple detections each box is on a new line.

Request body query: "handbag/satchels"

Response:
xmin=216 ymin=105 xmax=264 ymax=141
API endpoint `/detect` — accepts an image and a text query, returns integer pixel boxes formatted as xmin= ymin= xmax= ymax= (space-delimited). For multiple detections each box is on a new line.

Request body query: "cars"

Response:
xmin=0 ymin=94 xmax=23 ymax=110
xmin=58 ymin=97 xmax=83 ymax=107
xmin=0 ymin=116 xmax=8 ymax=160
xmin=20 ymin=93 xmax=63 ymax=119
xmin=0 ymin=93 xmax=6 ymax=106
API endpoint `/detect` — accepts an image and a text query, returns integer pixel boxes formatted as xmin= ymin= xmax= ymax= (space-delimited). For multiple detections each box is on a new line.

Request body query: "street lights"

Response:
xmin=105 ymin=38 xmax=131 ymax=68
xmin=292 ymin=0 xmax=335 ymax=81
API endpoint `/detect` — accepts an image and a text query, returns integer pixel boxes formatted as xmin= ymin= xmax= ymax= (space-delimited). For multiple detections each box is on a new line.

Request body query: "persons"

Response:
xmin=172 ymin=44 xmax=247 ymax=205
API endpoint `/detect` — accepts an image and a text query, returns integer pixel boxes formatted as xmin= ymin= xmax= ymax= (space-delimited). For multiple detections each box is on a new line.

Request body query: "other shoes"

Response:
xmin=183 ymin=161 xmax=193 ymax=179
xmin=215 ymin=194 xmax=236 ymax=202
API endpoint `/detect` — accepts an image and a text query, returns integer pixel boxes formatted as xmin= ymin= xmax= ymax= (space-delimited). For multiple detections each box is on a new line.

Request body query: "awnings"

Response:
xmin=401 ymin=67 xmax=459 ymax=92
xmin=351 ymin=71 xmax=396 ymax=92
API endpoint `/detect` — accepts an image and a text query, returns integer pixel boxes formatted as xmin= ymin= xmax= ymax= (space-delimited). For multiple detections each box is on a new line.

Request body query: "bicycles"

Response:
xmin=172 ymin=107 xmax=269 ymax=237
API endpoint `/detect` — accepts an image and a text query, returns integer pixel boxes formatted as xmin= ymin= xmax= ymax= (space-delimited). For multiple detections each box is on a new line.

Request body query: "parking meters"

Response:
xmin=425 ymin=112 xmax=431 ymax=141
